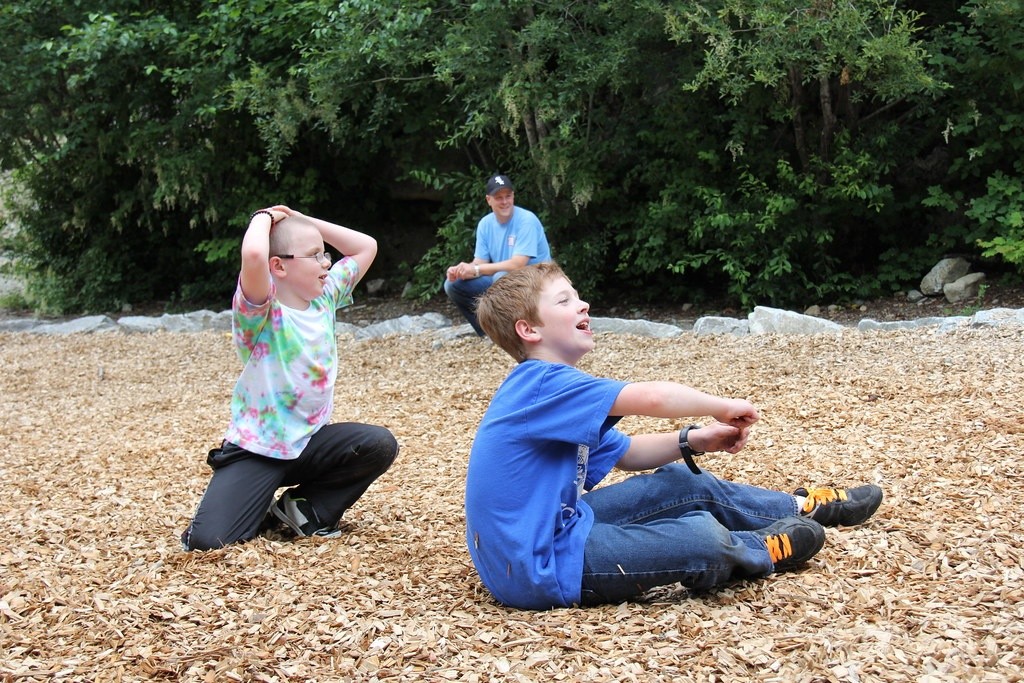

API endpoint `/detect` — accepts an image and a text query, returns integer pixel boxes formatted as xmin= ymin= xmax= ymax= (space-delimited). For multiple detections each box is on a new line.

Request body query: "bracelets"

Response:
xmin=678 ymin=423 xmax=707 ymax=476
xmin=474 ymin=263 xmax=480 ymax=278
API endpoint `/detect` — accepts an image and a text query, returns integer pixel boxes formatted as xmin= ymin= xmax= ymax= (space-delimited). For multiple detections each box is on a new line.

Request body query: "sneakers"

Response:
xmin=271 ymin=487 xmax=342 ymax=541
xmin=744 ymin=517 xmax=826 ymax=577
xmin=792 ymin=483 xmax=883 ymax=528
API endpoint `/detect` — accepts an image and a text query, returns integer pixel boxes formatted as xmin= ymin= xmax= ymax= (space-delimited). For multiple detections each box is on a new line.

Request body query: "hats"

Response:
xmin=486 ymin=175 xmax=514 ymax=196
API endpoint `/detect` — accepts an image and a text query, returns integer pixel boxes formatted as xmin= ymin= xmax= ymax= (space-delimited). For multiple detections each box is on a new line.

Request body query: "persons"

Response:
xmin=444 ymin=173 xmax=551 ymax=339
xmin=464 ymin=259 xmax=884 ymax=613
xmin=180 ymin=203 xmax=402 ymax=557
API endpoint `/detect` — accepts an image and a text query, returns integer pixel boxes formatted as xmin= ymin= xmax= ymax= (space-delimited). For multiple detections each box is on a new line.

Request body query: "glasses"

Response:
xmin=272 ymin=251 xmax=333 ymax=264
